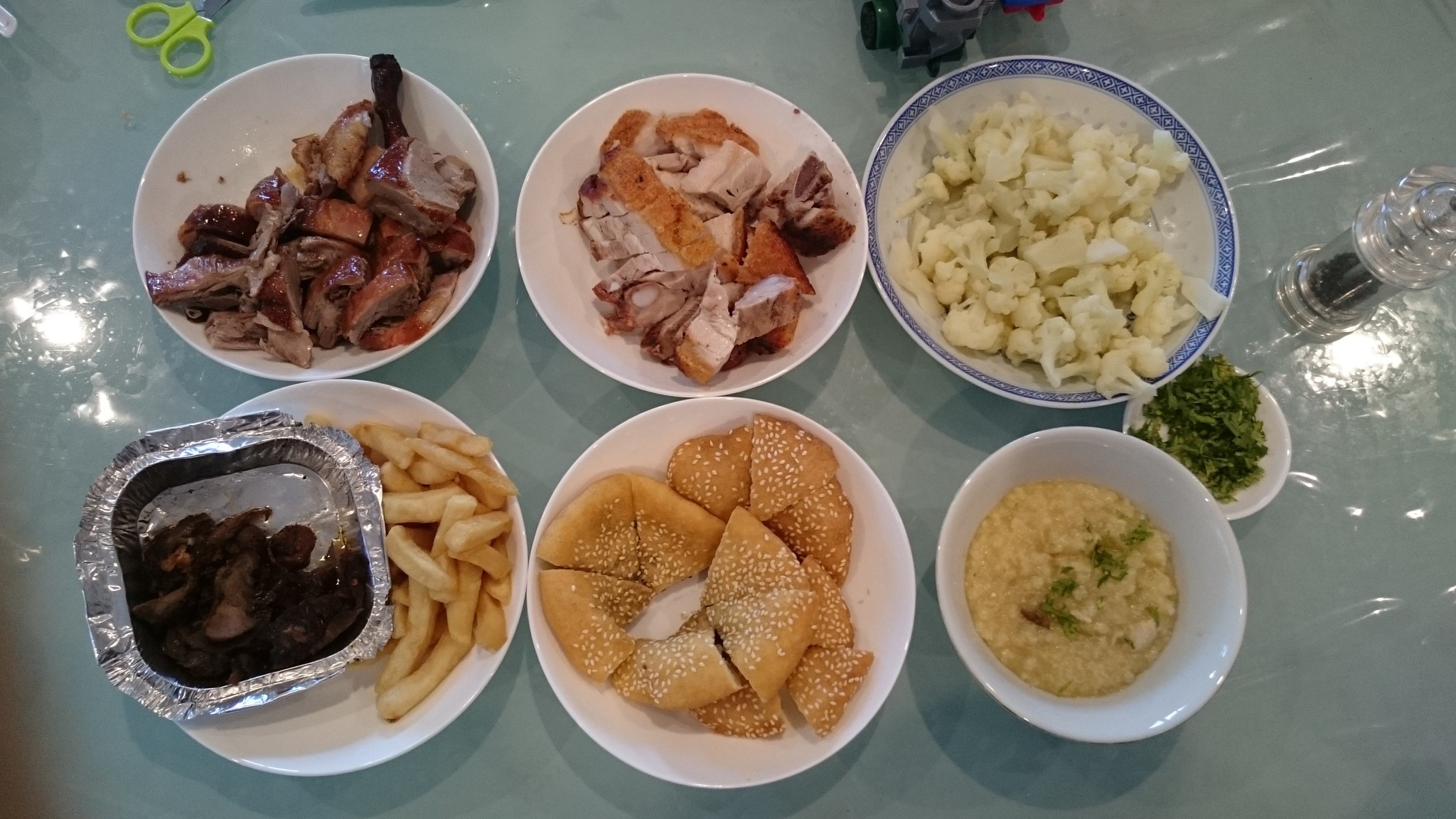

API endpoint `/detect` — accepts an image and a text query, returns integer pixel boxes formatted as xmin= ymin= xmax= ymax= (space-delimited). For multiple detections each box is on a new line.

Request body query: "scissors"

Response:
xmin=121 ymin=0 xmax=235 ymax=79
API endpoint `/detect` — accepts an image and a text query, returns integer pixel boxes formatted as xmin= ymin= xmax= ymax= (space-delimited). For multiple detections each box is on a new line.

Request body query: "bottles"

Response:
xmin=1274 ymin=165 xmax=1456 ymax=344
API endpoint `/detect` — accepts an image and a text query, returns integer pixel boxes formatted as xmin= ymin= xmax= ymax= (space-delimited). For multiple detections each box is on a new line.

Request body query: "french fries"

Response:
xmin=346 ymin=421 xmax=521 ymax=721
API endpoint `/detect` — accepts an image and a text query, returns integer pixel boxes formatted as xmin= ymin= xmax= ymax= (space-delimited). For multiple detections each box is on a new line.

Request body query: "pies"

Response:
xmin=536 ymin=415 xmax=876 ymax=742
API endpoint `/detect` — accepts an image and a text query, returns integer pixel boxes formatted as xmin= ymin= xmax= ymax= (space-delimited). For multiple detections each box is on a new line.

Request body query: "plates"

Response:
xmin=176 ymin=381 xmax=529 ymax=778
xmin=935 ymin=425 xmax=1248 ymax=743
xmin=1122 ymin=364 xmax=1291 ymax=520
xmin=864 ymin=56 xmax=1240 ymax=411
xmin=132 ymin=53 xmax=500 ymax=380
xmin=515 ymin=73 xmax=868 ymax=398
xmin=527 ymin=398 xmax=916 ymax=791
